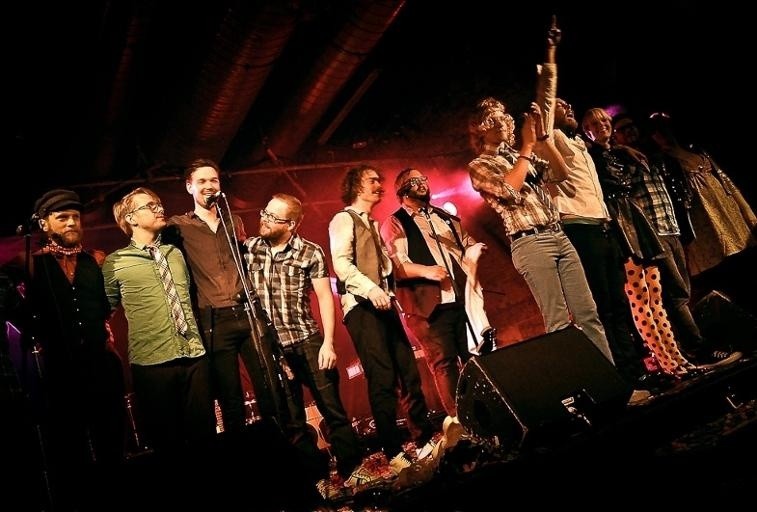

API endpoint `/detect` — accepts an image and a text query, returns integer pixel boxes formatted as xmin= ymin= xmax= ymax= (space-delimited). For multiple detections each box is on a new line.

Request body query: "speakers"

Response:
xmin=454 ymin=324 xmax=634 ymax=454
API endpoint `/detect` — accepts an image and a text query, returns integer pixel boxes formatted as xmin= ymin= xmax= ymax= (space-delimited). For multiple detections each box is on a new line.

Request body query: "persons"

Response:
xmin=19 ymin=159 xmax=361 ymax=499
xmin=328 ymin=165 xmax=497 ymax=459
xmin=449 ymin=15 xmax=756 ymax=407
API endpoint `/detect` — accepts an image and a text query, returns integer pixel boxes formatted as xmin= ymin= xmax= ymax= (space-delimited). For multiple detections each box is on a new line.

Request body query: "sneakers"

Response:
xmin=316 ymin=476 xmax=338 ymax=500
xmin=627 ymin=388 xmax=652 ymax=407
xmin=342 ymin=470 xmax=372 ymax=489
xmin=386 ymin=449 xmax=414 ymax=475
xmin=413 ymin=438 xmax=437 ymax=460
xmin=695 ymin=348 xmax=742 ymax=370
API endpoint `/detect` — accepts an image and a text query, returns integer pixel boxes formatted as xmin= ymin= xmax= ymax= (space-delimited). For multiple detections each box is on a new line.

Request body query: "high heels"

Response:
xmin=663 ymin=370 xmax=694 ymax=384
xmin=686 ymin=361 xmax=714 ymax=376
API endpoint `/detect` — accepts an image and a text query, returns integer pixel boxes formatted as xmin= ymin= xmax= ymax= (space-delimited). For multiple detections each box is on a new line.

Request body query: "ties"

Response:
xmin=145 ymin=241 xmax=190 ymax=338
xmin=500 ymin=150 xmax=554 ymax=210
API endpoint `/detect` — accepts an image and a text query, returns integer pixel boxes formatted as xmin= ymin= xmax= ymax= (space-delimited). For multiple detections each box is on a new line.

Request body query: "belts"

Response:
xmin=507 ymin=224 xmax=561 ymax=245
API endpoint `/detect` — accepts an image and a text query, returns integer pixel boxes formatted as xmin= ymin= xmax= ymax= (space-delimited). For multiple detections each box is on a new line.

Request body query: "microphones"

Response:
xmin=206 ymin=191 xmax=222 ymax=206
xmin=396 ymin=182 xmax=415 ymax=197
xmin=16 ymin=212 xmax=40 ymax=236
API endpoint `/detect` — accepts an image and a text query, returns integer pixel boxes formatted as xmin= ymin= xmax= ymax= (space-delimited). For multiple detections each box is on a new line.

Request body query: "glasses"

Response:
xmin=130 ymin=201 xmax=163 ymax=214
xmin=259 ymin=207 xmax=289 ymax=225
xmin=403 ymin=174 xmax=428 ymax=188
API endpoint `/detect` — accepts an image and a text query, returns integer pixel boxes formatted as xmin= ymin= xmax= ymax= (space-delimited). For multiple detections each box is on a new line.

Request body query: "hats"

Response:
xmin=33 ymin=188 xmax=82 ymax=220
